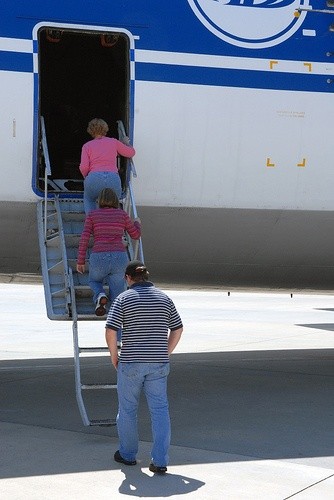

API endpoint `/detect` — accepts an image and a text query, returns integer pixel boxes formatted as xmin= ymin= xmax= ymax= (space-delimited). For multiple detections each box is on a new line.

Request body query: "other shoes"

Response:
xmin=114 ymin=450 xmax=136 ymax=465
xmin=149 ymin=463 xmax=167 ymax=471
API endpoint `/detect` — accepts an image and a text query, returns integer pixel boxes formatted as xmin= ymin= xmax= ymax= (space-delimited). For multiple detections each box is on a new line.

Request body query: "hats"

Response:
xmin=124 ymin=259 xmax=147 ymax=277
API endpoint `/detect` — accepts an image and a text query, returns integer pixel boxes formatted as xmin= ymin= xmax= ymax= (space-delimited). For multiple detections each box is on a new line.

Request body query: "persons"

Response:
xmin=105 ymin=261 xmax=183 ymax=473
xmin=79 ymin=118 xmax=136 ymax=215
xmin=76 ymin=188 xmax=142 ymax=350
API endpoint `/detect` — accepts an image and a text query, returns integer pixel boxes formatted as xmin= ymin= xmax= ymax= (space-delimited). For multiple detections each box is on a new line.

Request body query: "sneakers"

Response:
xmin=95 ymin=294 xmax=108 ymax=316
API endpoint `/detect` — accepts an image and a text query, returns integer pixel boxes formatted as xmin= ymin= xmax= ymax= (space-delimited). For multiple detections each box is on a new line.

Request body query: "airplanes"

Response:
xmin=1 ymin=0 xmax=334 ymax=320
